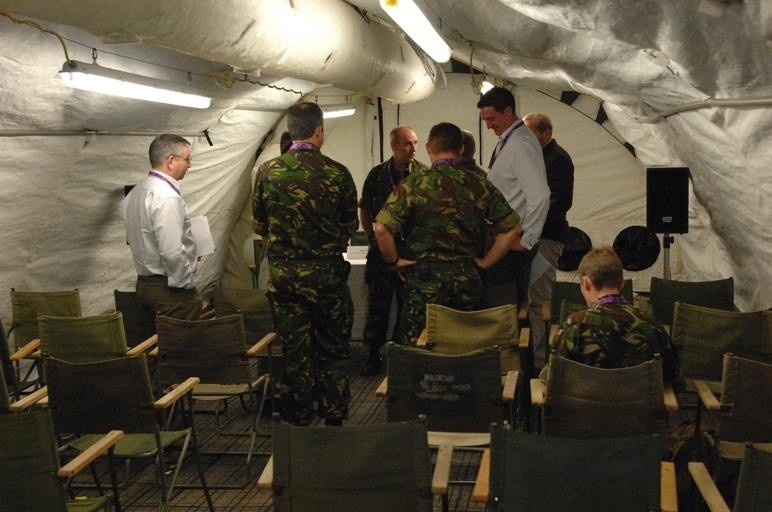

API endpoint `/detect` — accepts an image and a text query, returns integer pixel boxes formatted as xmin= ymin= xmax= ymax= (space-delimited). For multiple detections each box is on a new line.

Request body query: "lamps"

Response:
xmin=61 ymin=46 xmax=213 ymax=111
xmin=380 ymin=0 xmax=455 ymax=65
xmin=474 ymin=74 xmax=494 ymax=96
xmin=310 ymin=97 xmax=358 ymax=120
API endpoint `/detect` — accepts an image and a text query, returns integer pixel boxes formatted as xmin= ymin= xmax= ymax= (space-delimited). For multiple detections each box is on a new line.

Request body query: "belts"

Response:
xmin=137 ymin=274 xmax=168 ymax=281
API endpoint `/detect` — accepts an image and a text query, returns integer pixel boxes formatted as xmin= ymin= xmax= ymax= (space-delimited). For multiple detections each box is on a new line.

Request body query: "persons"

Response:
xmin=274 ymin=125 xmax=293 ymax=159
xmin=474 ymin=86 xmax=554 ymax=346
xmin=460 ymin=129 xmax=493 ymax=262
xmin=250 ymin=98 xmax=363 ymax=429
xmin=117 ymin=133 xmax=207 ymax=452
xmin=537 ymin=246 xmax=681 ymax=398
xmin=519 ymin=112 xmax=575 ymax=376
xmin=371 ymin=121 xmax=522 ymax=350
xmin=357 ymin=125 xmax=428 ymax=377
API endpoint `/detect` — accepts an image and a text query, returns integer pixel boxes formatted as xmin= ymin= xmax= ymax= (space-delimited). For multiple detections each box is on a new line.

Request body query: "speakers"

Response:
xmin=646 ymin=167 xmax=690 ymax=234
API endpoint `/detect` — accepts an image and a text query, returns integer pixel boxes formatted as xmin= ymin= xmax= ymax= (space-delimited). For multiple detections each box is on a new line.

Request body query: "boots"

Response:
xmin=369 ymin=334 xmax=388 ymax=369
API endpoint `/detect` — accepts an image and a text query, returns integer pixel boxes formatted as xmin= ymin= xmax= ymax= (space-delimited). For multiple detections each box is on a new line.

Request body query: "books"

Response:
xmin=187 ymin=210 xmax=216 ymax=265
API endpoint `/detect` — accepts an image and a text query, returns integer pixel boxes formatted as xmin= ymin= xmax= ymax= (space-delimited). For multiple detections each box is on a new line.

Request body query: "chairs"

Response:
xmin=689 ymin=350 xmax=772 ymax=461
xmin=1 ymin=365 xmax=119 ymax=512
xmin=0 ymin=283 xmax=161 ymax=416
xmin=42 ymin=349 xmax=215 ymax=512
xmin=155 ymin=310 xmax=279 ymax=490
xmin=254 ymin=411 xmax=454 ymax=512
xmin=469 ymin=424 xmax=681 ymax=512
xmin=211 ymin=286 xmax=323 ymax=415
xmin=686 ymin=439 xmax=771 ymax=512
xmin=416 ymin=274 xmax=772 ymax=398
xmin=529 ymin=348 xmax=680 ymax=438
xmin=374 ymin=340 xmax=525 ymax=432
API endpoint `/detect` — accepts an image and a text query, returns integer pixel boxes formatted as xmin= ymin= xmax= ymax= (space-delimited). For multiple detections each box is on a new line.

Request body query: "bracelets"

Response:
xmin=388 ymin=257 xmax=401 ymax=267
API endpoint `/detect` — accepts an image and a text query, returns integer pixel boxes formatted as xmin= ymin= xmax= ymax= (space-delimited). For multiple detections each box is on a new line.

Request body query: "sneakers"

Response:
xmin=295 ymin=416 xmax=314 ymax=427
xmin=324 ymin=416 xmax=344 ymax=426
